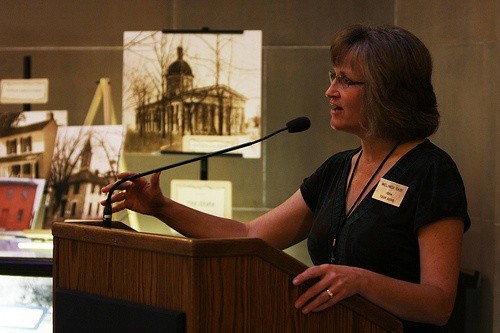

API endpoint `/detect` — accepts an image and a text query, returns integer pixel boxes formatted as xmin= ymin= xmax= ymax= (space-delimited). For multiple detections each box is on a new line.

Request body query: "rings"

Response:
xmin=327 ymin=289 xmax=333 ymax=298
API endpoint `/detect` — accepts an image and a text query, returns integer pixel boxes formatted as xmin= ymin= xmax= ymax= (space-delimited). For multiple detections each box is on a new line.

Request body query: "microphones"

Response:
xmin=102 ymin=116 xmax=311 ymax=227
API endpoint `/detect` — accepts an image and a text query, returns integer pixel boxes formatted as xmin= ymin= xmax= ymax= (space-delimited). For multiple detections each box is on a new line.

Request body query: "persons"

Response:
xmin=100 ymin=23 xmax=471 ymax=333
